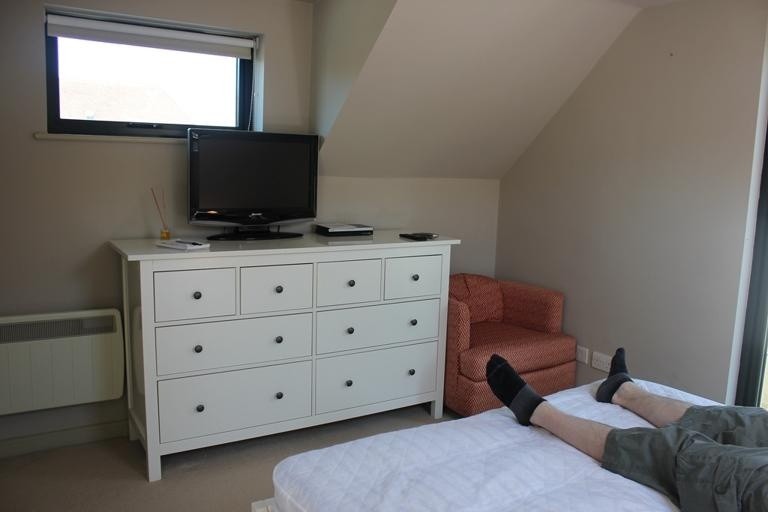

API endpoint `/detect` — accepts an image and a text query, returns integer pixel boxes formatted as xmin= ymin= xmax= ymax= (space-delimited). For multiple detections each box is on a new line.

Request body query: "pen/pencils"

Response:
xmin=175 ymin=240 xmax=202 ymax=246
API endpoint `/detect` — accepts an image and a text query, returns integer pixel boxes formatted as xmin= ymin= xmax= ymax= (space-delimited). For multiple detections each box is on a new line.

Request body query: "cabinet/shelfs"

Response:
xmin=110 ymin=228 xmax=463 ymax=482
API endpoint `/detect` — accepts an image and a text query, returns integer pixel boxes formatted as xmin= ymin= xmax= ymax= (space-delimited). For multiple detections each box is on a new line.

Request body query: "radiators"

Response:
xmin=0 ymin=308 xmax=124 ymax=417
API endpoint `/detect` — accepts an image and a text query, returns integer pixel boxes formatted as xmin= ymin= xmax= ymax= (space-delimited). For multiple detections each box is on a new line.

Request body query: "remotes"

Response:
xmin=399 ymin=233 xmax=427 ymax=241
xmin=415 ymin=232 xmax=439 ymax=239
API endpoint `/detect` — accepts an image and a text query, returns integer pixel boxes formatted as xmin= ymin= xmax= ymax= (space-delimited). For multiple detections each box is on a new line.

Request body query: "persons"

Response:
xmin=486 ymin=347 xmax=764 ymax=512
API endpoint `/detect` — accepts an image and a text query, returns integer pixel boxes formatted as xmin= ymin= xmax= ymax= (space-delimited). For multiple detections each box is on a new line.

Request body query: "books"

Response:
xmin=157 ymin=238 xmax=210 ymax=249
xmin=315 ymin=222 xmax=374 ymax=233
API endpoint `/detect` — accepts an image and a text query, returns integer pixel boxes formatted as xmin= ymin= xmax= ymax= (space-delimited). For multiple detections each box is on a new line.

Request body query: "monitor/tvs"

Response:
xmin=188 ymin=129 xmax=318 ymax=241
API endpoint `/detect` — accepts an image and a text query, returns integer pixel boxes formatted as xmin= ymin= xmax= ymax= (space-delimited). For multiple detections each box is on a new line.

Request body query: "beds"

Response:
xmin=443 ymin=273 xmax=577 ymax=417
xmin=248 ymin=375 xmax=734 ymax=512
